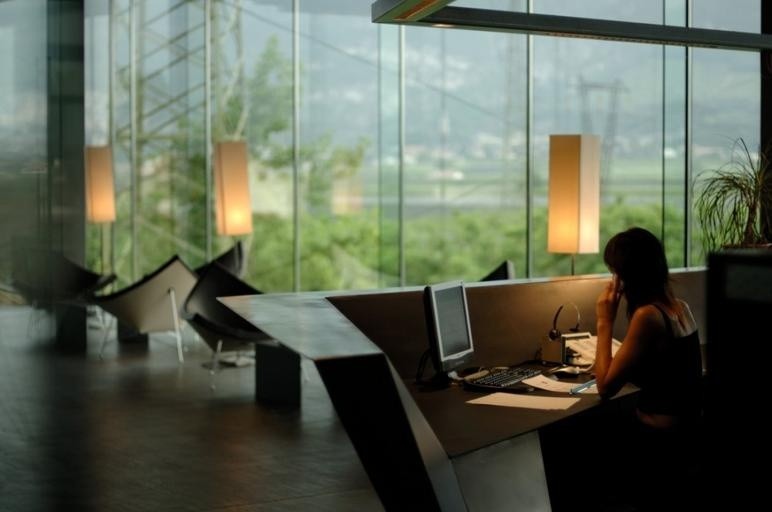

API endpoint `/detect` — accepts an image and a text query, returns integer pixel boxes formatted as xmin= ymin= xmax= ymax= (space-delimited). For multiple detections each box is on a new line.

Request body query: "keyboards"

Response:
xmin=467 ymin=367 xmax=542 ymax=389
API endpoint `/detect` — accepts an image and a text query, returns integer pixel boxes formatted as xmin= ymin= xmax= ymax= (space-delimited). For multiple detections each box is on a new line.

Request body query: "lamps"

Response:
xmin=544 ymin=134 xmax=599 ymax=278
xmin=210 ymin=134 xmax=256 ymax=367
xmin=83 ymin=141 xmax=116 ymax=329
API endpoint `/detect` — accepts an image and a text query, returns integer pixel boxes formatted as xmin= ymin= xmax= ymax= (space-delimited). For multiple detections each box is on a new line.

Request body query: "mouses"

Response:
xmin=552 ymin=367 xmax=580 ymax=379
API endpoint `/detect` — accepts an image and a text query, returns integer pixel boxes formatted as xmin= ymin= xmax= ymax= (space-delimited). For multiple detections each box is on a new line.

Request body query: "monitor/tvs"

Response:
xmin=415 ymin=280 xmax=475 ymax=386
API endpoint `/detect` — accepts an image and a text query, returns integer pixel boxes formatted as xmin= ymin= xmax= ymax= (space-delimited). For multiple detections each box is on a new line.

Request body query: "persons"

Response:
xmin=594 ymin=226 xmax=706 ymax=432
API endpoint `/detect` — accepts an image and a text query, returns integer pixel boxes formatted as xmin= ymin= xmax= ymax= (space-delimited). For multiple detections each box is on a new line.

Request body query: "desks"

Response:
xmin=395 ymin=331 xmax=706 ymax=512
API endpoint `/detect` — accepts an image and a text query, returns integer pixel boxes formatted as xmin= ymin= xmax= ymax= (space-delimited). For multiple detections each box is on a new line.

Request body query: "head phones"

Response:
xmin=548 ymin=302 xmax=580 ymax=340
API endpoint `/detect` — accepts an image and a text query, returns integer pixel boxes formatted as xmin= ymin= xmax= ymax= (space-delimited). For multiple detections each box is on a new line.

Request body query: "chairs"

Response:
xmin=10 ymin=240 xmax=266 ymax=395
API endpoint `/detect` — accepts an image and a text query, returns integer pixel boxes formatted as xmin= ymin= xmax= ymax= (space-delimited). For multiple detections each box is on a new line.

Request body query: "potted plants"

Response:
xmin=695 ymin=127 xmax=772 ymax=253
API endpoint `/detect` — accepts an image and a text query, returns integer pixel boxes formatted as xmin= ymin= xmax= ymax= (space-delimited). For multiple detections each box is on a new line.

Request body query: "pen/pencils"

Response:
xmin=568 ymin=378 xmax=597 ymax=396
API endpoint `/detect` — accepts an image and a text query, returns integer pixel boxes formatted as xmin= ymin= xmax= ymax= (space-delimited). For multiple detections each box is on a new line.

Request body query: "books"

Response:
xmin=566 ymin=332 xmax=624 ymax=367
